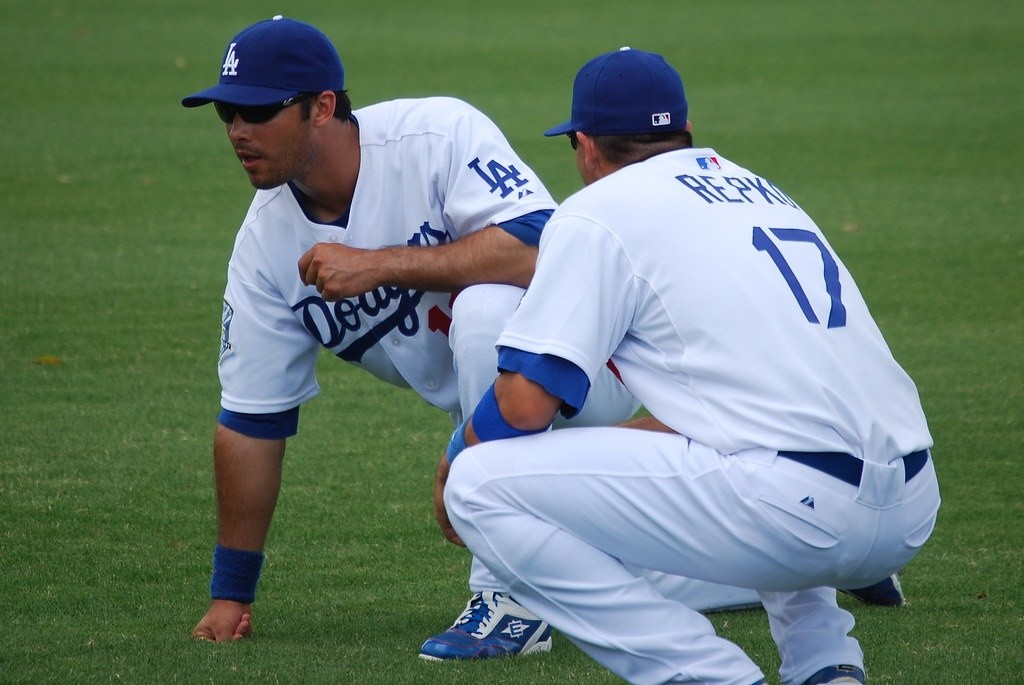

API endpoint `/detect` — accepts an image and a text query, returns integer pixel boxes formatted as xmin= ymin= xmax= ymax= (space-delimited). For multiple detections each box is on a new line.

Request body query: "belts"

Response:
xmin=777 ymin=450 xmax=928 ymax=489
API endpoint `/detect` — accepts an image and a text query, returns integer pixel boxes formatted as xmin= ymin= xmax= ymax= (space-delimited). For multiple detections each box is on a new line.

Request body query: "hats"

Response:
xmin=543 ymin=46 xmax=688 ymax=137
xmin=182 ymin=15 xmax=345 ymax=108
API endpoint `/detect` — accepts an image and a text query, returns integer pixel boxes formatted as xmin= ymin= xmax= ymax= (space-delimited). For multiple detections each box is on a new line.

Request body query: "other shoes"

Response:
xmin=802 ymin=663 xmax=865 ymax=685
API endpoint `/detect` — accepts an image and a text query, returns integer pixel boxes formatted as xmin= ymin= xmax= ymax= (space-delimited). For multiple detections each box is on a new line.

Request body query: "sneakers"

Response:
xmin=418 ymin=591 xmax=552 ymax=662
xmin=842 ymin=572 xmax=907 ymax=607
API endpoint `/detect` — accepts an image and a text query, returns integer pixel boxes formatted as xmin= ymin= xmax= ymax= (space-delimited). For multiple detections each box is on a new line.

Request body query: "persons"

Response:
xmin=182 ymin=15 xmax=904 ymax=661
xmin=418 ymin=46 xmax=943 ymax=685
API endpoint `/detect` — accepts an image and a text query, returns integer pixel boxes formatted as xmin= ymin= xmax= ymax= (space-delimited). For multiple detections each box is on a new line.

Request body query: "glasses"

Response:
xmin=214 ymin=93 xmax=318 ymax=124
xmin=567 ymin=133 xmax=576 ymax=150
